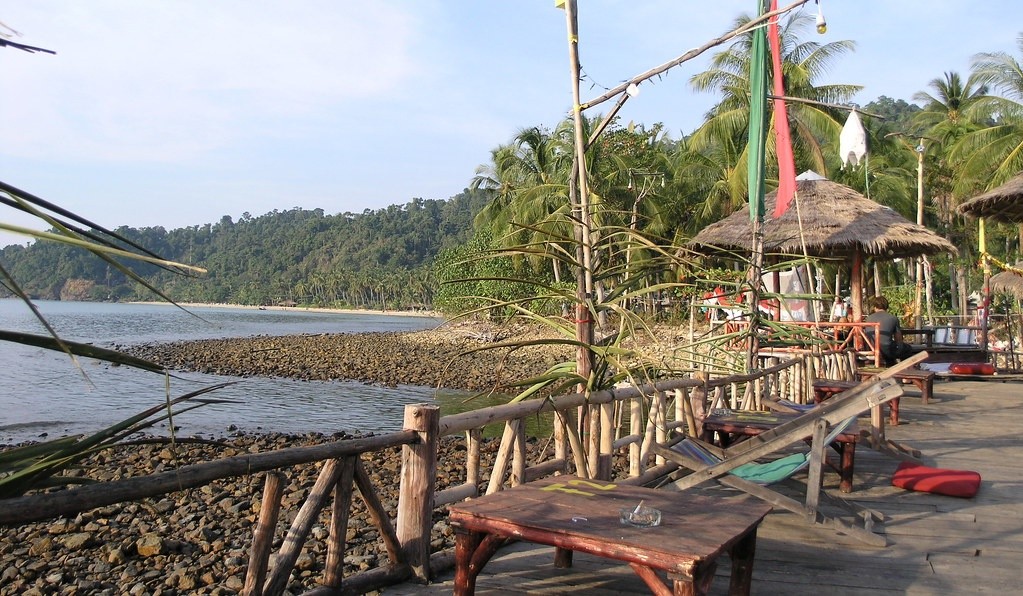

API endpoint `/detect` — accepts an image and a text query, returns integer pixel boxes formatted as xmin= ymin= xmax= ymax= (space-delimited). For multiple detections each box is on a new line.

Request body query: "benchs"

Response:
xmin=726 ymin=320 xmax=886 ymax=368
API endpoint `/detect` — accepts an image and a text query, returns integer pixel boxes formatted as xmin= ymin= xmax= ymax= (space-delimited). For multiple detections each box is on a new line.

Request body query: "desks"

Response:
xmin=856 ymin=368 xmax=935 ymax=404
xmin=812 ymin=381 xmax=900 ymax=426
xmin=447 ymin=475 xmax=774 ymax=596
xmin=702 ymin=409 xmax=861 ymax=493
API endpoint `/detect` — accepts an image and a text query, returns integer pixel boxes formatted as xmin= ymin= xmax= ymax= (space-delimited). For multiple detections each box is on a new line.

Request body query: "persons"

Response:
xmin=829 ymin=294 xmax=907 ymax=423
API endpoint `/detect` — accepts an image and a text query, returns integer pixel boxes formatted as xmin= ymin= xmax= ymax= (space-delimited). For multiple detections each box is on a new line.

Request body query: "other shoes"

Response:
xmin=903 ymin=379 xmax=912 ymax=383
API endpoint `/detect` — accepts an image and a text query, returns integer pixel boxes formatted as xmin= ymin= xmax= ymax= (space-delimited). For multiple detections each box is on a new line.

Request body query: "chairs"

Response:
xmin=762 ymin=351 xmax=929 ymax=465
xmin=651 ymin=380 xmax=905 ymax=547
